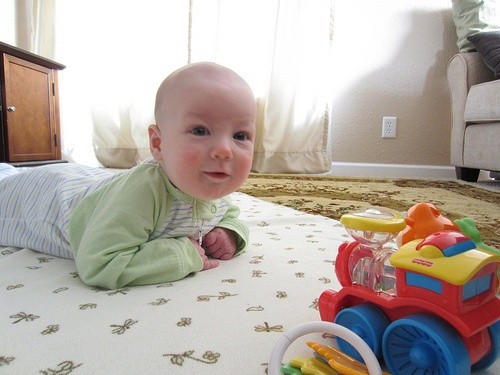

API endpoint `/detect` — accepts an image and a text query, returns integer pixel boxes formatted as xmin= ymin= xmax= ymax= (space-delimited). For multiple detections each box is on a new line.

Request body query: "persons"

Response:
xmin=0 ymin=62 xmax=257 ymax=289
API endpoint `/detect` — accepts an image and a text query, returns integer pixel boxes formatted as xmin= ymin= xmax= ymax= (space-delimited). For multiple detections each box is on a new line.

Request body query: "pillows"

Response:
xmin=465 ymin=30 xmax=500 ymax=80
xmin=449 ymin=0 xmax=500 ymax=52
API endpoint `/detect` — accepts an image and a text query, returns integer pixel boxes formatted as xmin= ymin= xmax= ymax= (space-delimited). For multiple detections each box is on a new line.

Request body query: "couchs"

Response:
xmin=446 ymin=51 xmax=500 ymax=183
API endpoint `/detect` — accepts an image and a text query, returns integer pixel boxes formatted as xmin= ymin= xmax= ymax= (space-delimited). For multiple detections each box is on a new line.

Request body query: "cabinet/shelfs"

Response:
xmin=0 ymin=40 xmax=65 ymax=162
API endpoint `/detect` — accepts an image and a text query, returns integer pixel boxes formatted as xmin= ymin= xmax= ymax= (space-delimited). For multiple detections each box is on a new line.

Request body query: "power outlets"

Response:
xmin=381 ymin=117 xmax=397 ymax=139
